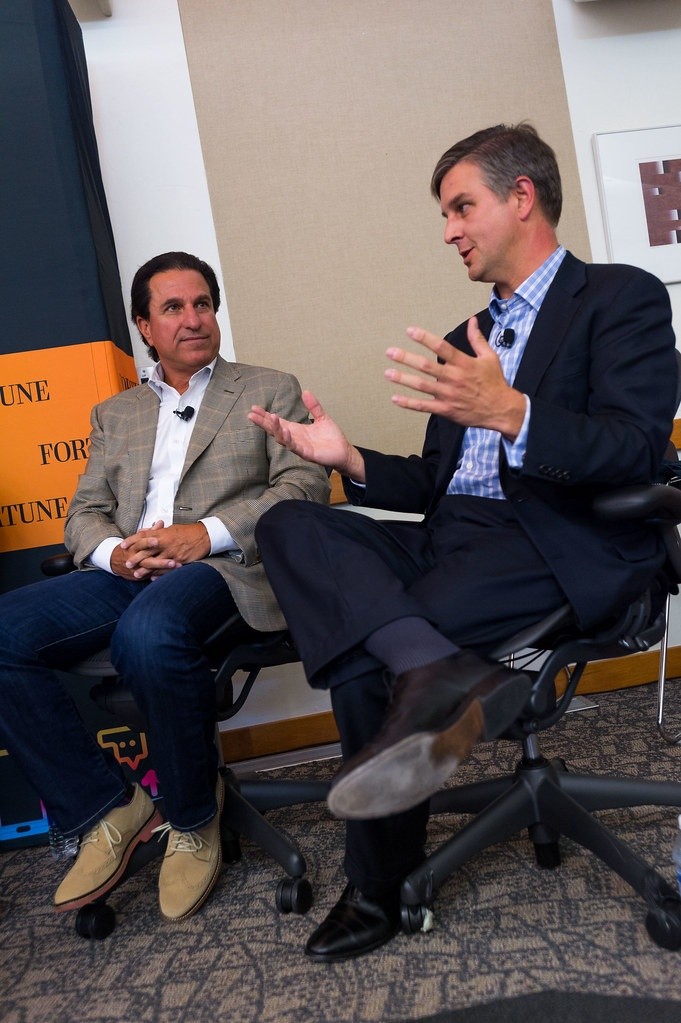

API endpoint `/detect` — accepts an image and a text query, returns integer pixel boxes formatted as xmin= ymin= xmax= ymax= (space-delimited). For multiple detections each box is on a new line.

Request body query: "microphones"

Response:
xmin=173 ymin=406 xmax=194 ymax=420
xmin=499 ymin=329 xmax=514 ymax=348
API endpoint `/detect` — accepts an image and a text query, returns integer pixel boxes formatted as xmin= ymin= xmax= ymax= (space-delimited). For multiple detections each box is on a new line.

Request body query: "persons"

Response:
xmin=0 ymin=250 xmax=340 ymax=930
xmin=245 ymin=123 xmax=680 ymax=964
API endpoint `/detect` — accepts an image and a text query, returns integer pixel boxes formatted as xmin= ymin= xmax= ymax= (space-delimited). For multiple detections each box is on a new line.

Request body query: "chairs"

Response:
xmin=322 ymin=437 xmax=680 ymax=954
xmin=41 ymin=419 xmax=345 ymax=940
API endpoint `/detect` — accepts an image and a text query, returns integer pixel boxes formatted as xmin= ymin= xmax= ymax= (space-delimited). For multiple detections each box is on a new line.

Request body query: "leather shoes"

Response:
xmin=306 ymin=878 xmax=399 ymax=962
xmin=157 ymin=773 xmax=224 ymax=921
xmin=325 ymin=648 xmax=532 ymax=821
xmin=52 ymin=782 xmax=162 ymax=913
